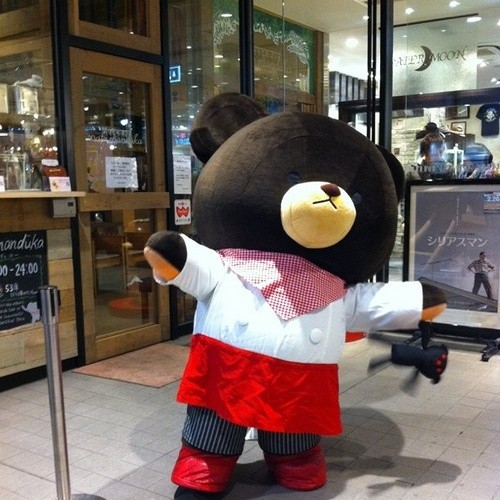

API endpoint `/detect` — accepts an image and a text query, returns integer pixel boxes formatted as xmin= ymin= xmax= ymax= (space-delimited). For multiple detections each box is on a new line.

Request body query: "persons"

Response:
xmin=468 ymin=251 xmax=494 ymax=299
xmin=409 ymin=123 xmax=454 ymax=182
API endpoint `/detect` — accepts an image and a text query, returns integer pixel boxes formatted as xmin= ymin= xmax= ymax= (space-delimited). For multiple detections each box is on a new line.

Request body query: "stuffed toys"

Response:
xmin=143 ymin=93 xmax=447 ymax=499
xmin=364 ymin=320 xmax=447 ymax=394
